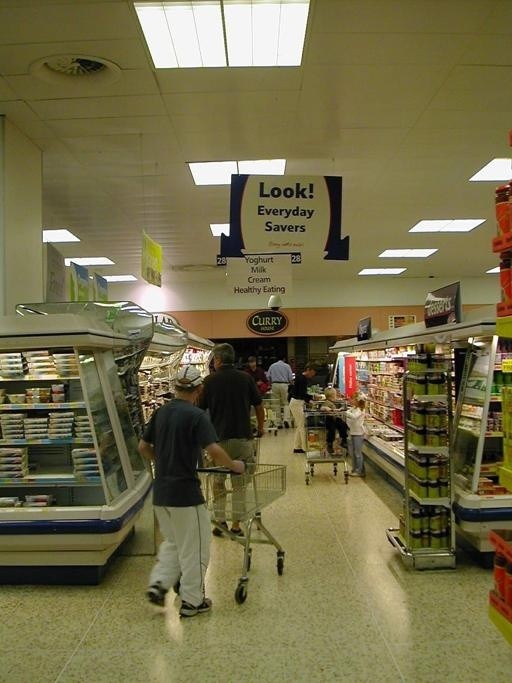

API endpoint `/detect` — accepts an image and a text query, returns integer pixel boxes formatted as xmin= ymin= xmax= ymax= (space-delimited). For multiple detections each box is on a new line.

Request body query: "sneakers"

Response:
xmin=147 ymin=582 xmax=166 ymax=607
xmin=230 ymin=528 xmax=244 ymax=540
xmin=293 ymin=449 xmax=306 ymax=454
xmin=348 ymin=470 xmax=366 ymax=477
xmin=178 ymin=596 xmax=212 ymax=617
xmin=212 ymin=523 xmax=228 ymax=535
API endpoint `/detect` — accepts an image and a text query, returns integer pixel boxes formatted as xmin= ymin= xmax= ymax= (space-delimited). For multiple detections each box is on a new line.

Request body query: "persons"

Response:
xmin=288 ymin=361 xmax=320 ymax=453
xmin=339 ymin=389 xmax=369 ymax=477
xmin=135 ymin=364 xmax=246 ymax=616
xmin=243 ymin=354 xmax=272 ymax=395
xmin=267 ymin=352 xmax=295 ymax=428
xmin=197 ymin=342 xmax=267 ymax=540
xmin=320 ymin=385 xmax=350 ymax=453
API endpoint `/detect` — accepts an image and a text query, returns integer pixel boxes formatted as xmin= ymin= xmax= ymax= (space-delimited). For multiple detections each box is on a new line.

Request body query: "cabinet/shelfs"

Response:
xmin=486 ymin=226 xmax=512 ymax=648
xmin=0 ymin=313 xmax=153 ymax=585
xmin=327 ymin=319 xmax=511 ymax=554
xmin=17 ymin=299 xmax=218 ymax=475
xmin=386 ymin=349 xmax=463 ymax=572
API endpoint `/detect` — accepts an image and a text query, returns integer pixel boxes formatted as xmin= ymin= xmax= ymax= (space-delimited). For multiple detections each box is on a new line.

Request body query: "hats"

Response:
xmin=174 ymin=364 xmax=203 ymax=388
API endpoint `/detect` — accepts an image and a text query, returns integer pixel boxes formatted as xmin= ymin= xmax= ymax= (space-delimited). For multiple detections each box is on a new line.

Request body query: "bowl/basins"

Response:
xmin=1 ymin=384 xmax=67 ymax=402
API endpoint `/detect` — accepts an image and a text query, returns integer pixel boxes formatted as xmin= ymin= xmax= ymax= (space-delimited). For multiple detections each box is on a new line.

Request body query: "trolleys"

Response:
xmin=300 ymin=396 xmax=351 ymax=486
xmin=252 ymin=396 xmax=283 ymax=437
xmin=191 ymin=459 xmax=290 ymax=608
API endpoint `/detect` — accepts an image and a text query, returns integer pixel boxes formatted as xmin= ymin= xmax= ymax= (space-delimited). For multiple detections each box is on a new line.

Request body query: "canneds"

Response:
xmin=405 ymin=355 xmax=450 ymax=548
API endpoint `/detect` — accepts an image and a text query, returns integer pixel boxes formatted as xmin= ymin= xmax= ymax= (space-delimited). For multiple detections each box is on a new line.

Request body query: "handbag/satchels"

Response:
xmin=288 ymin=384 xmax=294 ymax=403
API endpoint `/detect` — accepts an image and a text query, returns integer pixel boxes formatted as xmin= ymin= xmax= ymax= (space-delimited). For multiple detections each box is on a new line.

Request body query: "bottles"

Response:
xmin=398 ymin=353 xmax=451 ymax=550
